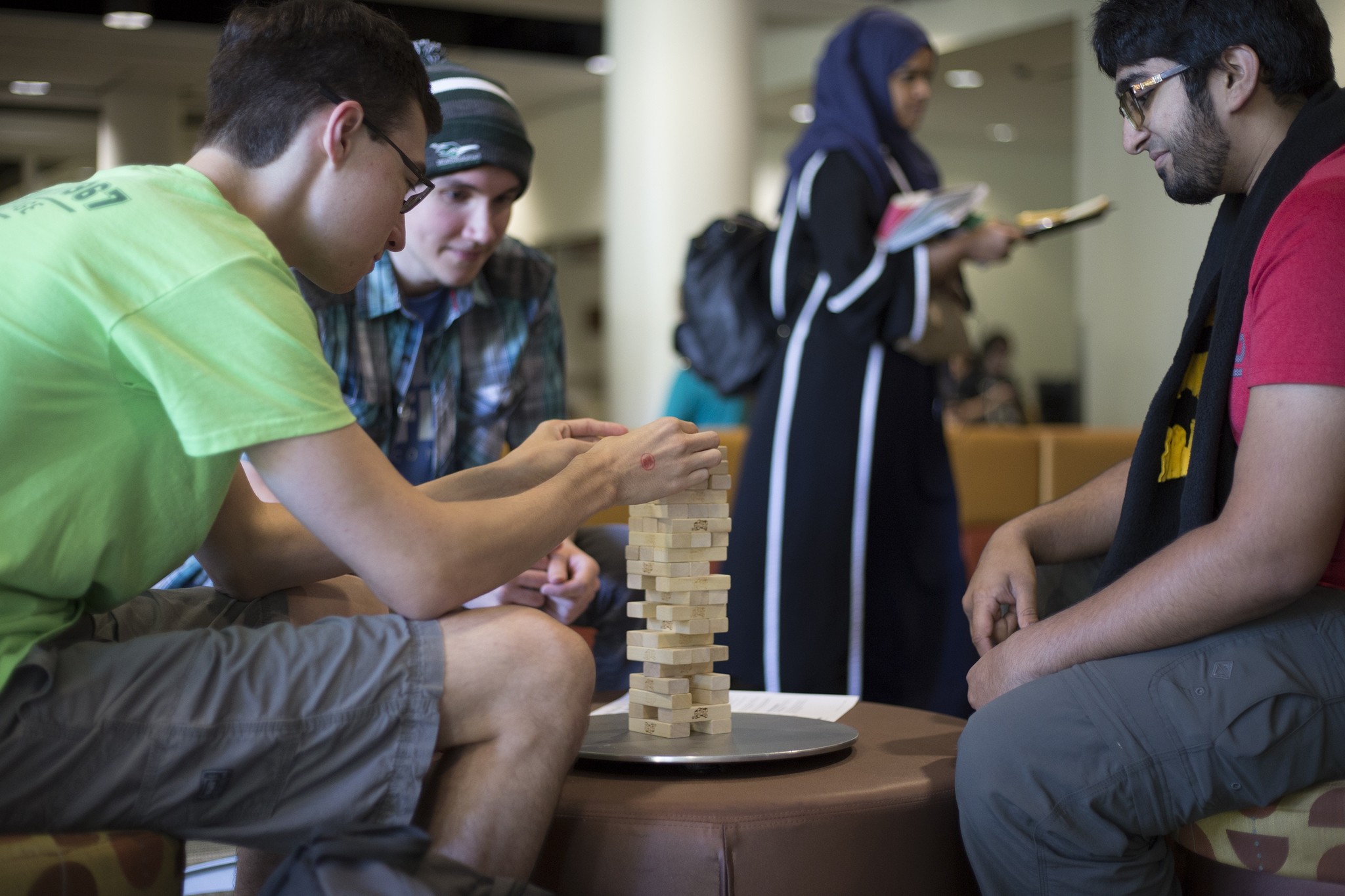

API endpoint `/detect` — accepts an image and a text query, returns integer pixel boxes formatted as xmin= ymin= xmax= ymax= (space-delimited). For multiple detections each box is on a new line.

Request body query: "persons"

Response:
xmin=0 ymin=2 xmax=723 ymax=896
xmin=662 ymin=264 xmax=1026 ymax=434
xmin=714 ymin=11 xmax=1021 ymax=720
xmin=160 ymin=37 xmax=647 ymax=702
xmin=953 ymin=0 xmax=1345 ymax=896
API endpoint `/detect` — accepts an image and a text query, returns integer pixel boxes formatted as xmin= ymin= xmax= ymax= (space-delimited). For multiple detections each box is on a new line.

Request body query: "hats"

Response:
xmin=417 ymin=63 xmax=534 ymax=201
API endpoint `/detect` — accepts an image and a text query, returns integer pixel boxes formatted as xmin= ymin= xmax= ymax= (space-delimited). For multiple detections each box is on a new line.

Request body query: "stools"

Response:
xmin=0 ymin=832 xmax=187 ymax=896
xmin=536 ymin=690 xmax=974 ymax=895
xmin=1174 ymin=778 xmax=1345 ymax=896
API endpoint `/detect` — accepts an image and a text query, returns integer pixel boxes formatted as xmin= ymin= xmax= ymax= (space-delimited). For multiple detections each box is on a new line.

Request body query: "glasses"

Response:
xmin=1118 ymin=62 xmax=1192 ymax=131
xmin=317 ymin=84 xmax=435 ymax=214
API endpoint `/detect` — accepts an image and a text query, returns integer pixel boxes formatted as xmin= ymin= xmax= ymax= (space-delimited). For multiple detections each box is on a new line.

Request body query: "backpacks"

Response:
xmin=678 ymin=216 xmax=777 ymax=399
